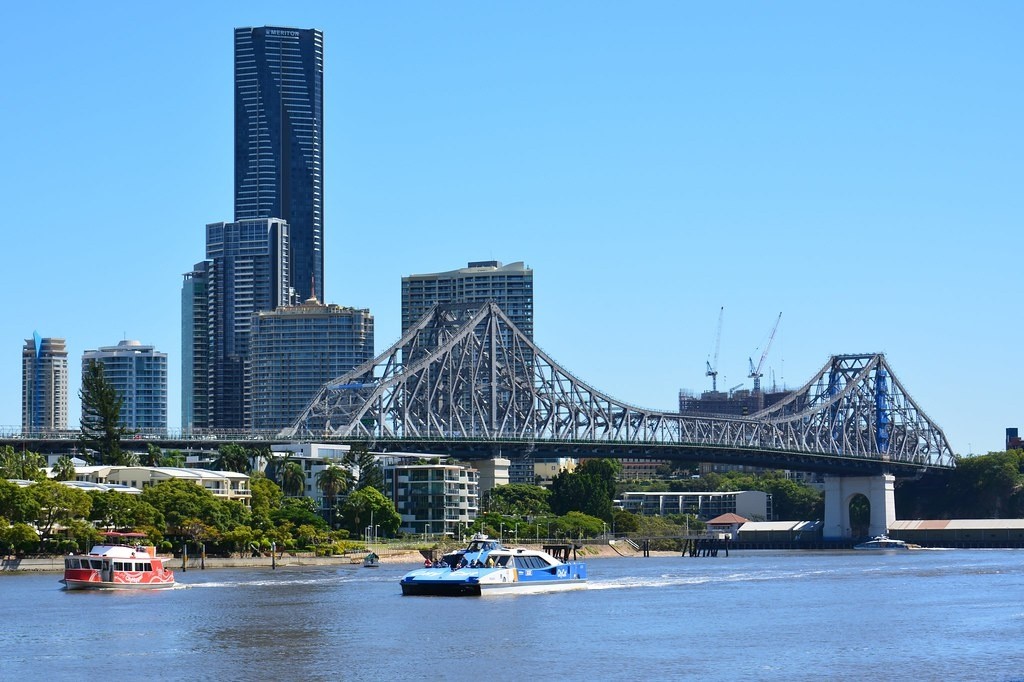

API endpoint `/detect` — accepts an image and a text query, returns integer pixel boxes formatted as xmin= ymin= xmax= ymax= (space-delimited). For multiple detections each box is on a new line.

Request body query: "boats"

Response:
xmin=362 ymin=552 xmax=380 ymax=567
xmin=398 ymin=533 xmax=590 ymax=596
xmin=59 ymin=531 xmax=176 ymax=590
xmin=852 ymin=531 xmax=922 ymax=550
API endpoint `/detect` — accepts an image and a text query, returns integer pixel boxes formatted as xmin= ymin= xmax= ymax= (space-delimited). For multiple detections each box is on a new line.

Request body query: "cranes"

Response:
xmin=704 ymin=306 xmax=727 ymax=391
xmin=747 ymin=313 xmax=783 ymax=414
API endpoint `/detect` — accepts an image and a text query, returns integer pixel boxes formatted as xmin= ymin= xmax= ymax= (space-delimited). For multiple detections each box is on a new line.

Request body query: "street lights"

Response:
xmin=499 ymin=522 xmax=505 ymax=544
xmin=365 ymin=527 xmax=370 ymax=549
xmin=602 ymin=522 xmax=607 ymax=545
xmin=458 ymin=523 xmax=464 ymax=543
xmin=548 ymin=522 xmax=551 ymax=544
xmin=536 ymin=524 xmax=542 ymax=543
xmin=481 ymin=522 xmax=485 ymax=539
xmin=367 ymin=525 xmax=373 ymax=553
xmin=515 ymin=522 xmax=520 ymax=545
xmin=424 ymin=523 xmax=430 ymax=546
xmin=375 ymin=525 xmax=380 ymax=553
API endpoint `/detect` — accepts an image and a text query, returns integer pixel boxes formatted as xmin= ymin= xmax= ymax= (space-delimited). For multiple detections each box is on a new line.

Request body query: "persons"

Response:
xmin=470 ymin=543 xmax=480 ymax=550
xmin=487 ymin=555 xmax=494 ymax=568
xmin=425 ymin=554 xmax=448 ymax=568
xmin=129 ymin=552 xmax=136 ymax=559
xmin=451 ymin=556 xmax=485 ymax=571
xmin=164 ymin=567 xmax=168 ymax=572
